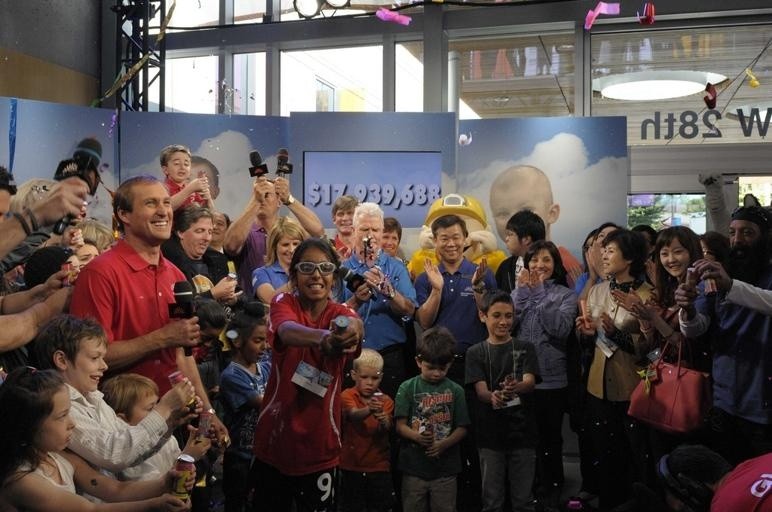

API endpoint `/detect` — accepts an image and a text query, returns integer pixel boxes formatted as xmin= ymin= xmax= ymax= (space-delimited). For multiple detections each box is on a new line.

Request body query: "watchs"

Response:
xmin=286 ymin=195 xmax=294 ymax=205
xmin=387 ymin=287 xmax=395 ymax=299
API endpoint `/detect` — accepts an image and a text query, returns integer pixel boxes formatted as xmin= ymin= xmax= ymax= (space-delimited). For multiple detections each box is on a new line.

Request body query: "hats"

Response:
xmin=24 ymin=246 xmax=74 ymax=289
xmin=710 ymin=453 xmax=772 ymax=512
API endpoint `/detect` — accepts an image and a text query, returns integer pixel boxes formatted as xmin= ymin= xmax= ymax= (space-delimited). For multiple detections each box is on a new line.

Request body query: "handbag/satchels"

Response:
xmin=627 ymin=359 xmax=711 ymax=435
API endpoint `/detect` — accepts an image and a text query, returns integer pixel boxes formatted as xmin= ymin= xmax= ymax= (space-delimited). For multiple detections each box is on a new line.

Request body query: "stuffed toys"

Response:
xmin=406 ymin=194 xmax=507 ymax=280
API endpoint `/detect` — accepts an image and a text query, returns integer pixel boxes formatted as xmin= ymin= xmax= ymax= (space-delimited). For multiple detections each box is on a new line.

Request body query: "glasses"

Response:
xmin=295 ymin=262 xmax=337 ymax=275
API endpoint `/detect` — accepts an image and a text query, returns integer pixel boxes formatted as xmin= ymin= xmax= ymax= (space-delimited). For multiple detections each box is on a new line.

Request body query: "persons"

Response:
xmin=0 ymin=145 xmax=769 ymax=512
xmin=490 ymin=165 xmax=585 ymax=289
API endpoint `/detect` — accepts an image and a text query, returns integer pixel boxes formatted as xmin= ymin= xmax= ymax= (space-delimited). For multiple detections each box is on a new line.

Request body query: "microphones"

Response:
xmin=53 ymin=137 xmax=103 ymax=237
xmin=248 ymin=150 xmax=269 ymax=198
xmin=277 ymin=148 xmax=294 ymax=181
xmin=339 ymin=267 xmax=379 ymax=303
xmin=173 ymin=280 xmax=194 ymax=358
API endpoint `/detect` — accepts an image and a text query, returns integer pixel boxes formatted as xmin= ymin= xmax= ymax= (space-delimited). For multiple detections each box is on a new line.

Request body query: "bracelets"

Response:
xmin=474 ymin=286 xmax=486 ymax=294
xmin=14 ymin=211 xmax=32 ymax=236
xmin=202 ymin=408 xmax=216 ymax=416
xmin=25 ymin=207 xmax=39 ymax=231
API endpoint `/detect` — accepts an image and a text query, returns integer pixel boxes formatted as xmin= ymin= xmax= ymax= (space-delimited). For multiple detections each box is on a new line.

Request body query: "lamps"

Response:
xmin=590 ymin=70 xmax=730 ymax=101
